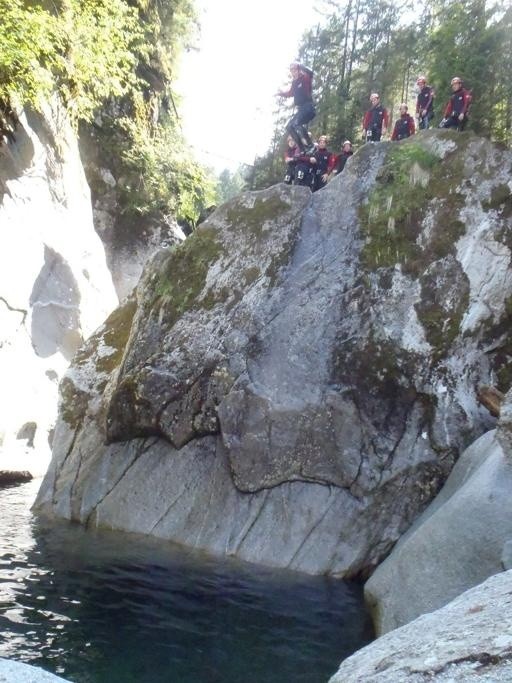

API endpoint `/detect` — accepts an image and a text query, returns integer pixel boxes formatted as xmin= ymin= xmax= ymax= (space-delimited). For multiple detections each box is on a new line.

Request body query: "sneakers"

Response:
xmin=298 ymin=146 xmax=319 ymax=157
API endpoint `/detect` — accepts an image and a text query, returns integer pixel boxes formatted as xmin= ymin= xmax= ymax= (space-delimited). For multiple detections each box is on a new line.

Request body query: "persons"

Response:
xmin=360 ymin=90 xmax=390 ymax=141
xmin=390 ymin=101 xmax=417 ymax=139
xmin=414 ymin=75 xmax=435 ymax=129
xmin=437 ymin=76 xmax=474 ymax=133
xmin=282 ymin=130 xmax=355 ymax=194
xmin=274 ymin=60 xmax=318 ymax=157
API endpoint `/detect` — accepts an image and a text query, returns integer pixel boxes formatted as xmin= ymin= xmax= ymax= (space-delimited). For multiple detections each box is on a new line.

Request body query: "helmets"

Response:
xmin=450 ymin=76 xmax=464 ymax=85
xmin=370 ymin=93 xmax=379 ymax=100
xmin=416 ymin=76 xmax=427 ymax=85
xmin=284 ymin=131 xmax=354 ymax=146
